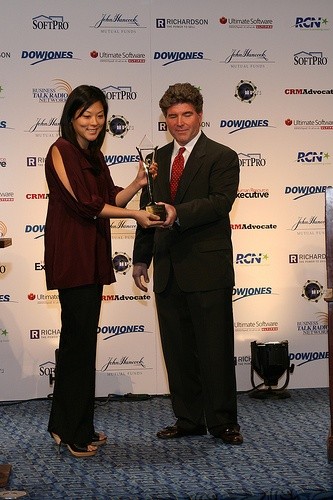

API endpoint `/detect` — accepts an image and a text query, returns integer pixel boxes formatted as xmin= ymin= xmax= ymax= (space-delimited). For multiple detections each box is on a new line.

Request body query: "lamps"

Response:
xmin=248 ymin=340 xmax=294 ymax=400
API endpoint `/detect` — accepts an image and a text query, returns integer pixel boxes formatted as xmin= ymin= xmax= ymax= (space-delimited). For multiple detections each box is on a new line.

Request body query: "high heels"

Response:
xmin=50 ymin=428 xmax=108 ymax=457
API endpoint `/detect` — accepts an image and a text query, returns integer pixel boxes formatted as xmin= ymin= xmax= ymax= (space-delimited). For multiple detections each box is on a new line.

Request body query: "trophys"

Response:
xmin=136 ymin=133 xmax=166 ymax=223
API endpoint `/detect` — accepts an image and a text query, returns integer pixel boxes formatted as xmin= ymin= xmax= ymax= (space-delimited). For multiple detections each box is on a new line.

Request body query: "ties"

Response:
xmin=170 ymin=147 xmax=186 ymax=206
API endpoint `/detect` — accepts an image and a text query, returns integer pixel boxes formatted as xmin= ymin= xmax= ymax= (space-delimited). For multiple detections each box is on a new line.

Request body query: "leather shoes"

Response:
xmin=158 ymin=423 xmax=206 ymax=440
xmin=221 ymin=425 xmax=242 ymax=446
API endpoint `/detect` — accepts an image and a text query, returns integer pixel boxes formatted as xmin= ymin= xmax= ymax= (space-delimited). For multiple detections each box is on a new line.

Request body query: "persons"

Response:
xmin=132 ymin=83 xmax=244 ymax=446
xmin=43 ymin=85 xmax=165 ymax=457
